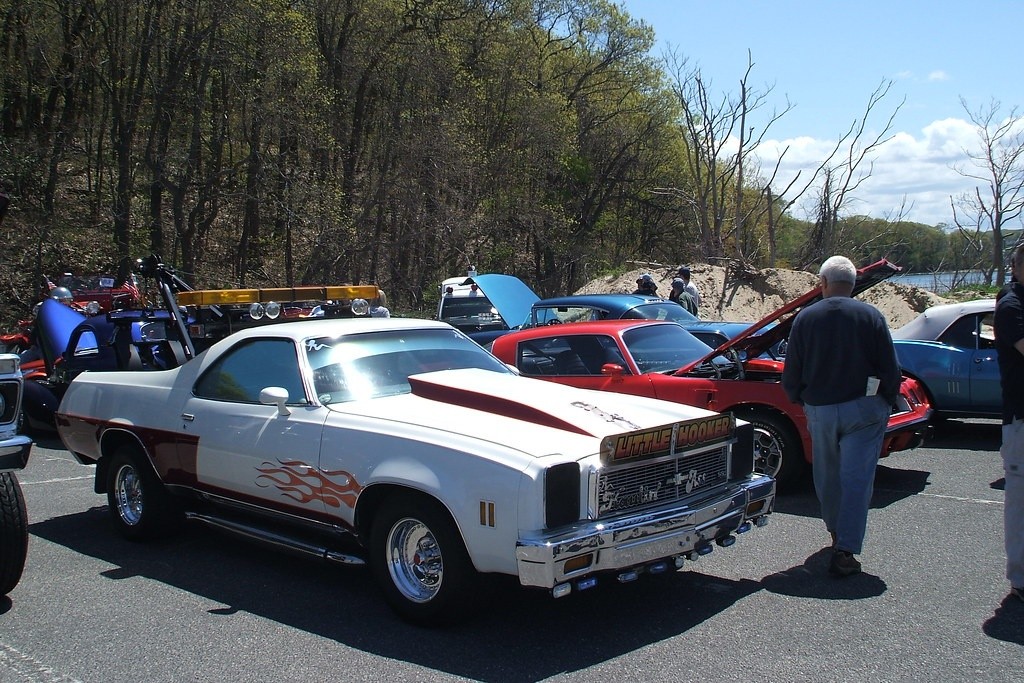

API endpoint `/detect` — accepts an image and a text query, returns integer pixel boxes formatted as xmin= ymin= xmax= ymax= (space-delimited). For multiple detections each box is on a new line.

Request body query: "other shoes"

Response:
xmin=1011 ymin=586 xmax=1024 ymax=602
xmin=991 ymin=478 xmax=1005 ymax=489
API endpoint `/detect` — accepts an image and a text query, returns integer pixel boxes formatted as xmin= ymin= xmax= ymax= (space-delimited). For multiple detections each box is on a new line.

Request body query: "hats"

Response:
xmin=636 ymin=274 xmax=651 ymax=283
xmin=671 ymin=278 xmax=684 ymax=286
xmin=675 ymin=268 xmax=690 ymax=276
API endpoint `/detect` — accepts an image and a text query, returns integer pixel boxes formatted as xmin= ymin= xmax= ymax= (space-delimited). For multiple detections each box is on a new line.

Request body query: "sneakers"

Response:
xmin=828 ymin=552 xmax=862 ymax=575
xmin=831 ymin=531 xmax=837 ymax=548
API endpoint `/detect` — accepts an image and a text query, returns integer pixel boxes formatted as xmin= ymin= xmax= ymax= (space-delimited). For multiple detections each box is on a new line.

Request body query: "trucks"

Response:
xmin=438 ymin=276 xmax=505 ymax=334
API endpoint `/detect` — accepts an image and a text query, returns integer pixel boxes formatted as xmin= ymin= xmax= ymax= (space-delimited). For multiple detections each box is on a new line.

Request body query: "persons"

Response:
xmin=631 ymin=274 xmax=662 ymax=319
xmin=665 ymin=267 xmax=702 ymax=319
xmin=992 ymin=243 xmax=1024 ymax=622
xmin=781 ymin=255 xmax=901 ymax=577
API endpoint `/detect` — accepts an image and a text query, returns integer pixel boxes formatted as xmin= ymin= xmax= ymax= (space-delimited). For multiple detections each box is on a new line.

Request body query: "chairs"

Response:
xmin=556 ymin=344 xmax=633 ymax=377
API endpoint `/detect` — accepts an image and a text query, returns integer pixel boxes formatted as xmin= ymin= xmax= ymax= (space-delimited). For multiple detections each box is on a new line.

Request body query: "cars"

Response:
xmin=461 ymin=274 xmax=794 ymax=360
xmin=879 ymin=296 xmax=1007 ymax=419
xmin=51 ymin=286 xmax=776 ymax=628
xmin=461 ymin=260 xmax=935 ymax=491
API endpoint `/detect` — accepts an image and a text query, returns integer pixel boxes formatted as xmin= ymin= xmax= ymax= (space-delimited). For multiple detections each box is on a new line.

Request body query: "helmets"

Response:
xmin=49 ymin=287 xmax=74 ymax=299
